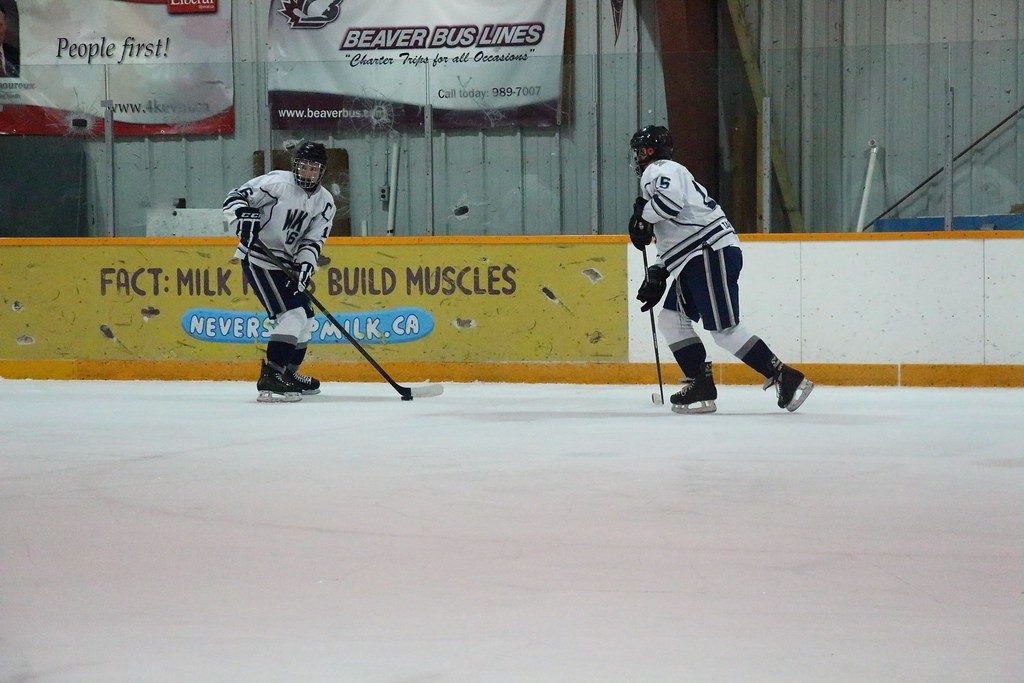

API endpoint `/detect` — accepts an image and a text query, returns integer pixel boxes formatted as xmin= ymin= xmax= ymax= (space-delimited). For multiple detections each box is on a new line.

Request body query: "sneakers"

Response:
xmin=284 ymin=368 xmax=321 ymax=395
xmin=762 ymin=354 xmax=814 ymax=411
xmin=670 ymin=361 xmax=717 ymax=413
xmin=257 ymin=359 xmax=302 ymax=402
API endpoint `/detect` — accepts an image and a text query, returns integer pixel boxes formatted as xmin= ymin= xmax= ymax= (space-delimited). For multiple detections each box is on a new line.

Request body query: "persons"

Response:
xmin=223 ymin=141 xmax=337 ymax=403
xmin=629 ymin=124 xmax=815 ymax=415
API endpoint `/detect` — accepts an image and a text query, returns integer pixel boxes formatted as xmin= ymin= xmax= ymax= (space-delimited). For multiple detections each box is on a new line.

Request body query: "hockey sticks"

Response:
xmin=255 ymin=238 xmax=444 ymax=398
xmin=639 ymin=222 xmax=664 ymax=405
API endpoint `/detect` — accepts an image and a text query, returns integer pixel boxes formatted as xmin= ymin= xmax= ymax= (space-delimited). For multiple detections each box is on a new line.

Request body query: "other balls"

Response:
xmin=400 ymin=395 xmax=413 ymax=400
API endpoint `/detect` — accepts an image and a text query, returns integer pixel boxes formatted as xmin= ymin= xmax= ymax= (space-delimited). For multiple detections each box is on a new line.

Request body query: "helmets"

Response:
xmin=293 ymin=140 xmax=328 ymax=189
xmin=629 ymin=125 xmax=674 ymax=179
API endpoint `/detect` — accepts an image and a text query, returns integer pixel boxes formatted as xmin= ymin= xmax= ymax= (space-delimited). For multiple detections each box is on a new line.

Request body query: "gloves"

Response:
xmin=235 ymin=207 xmax=260 ymax=249
xmin=285 ymin=261 xmax=315 ymax=296
xmin=629 ymin=196 xmax=653 ymax=251
xmin=636 ymin=266 xmax=671 ymax=312
